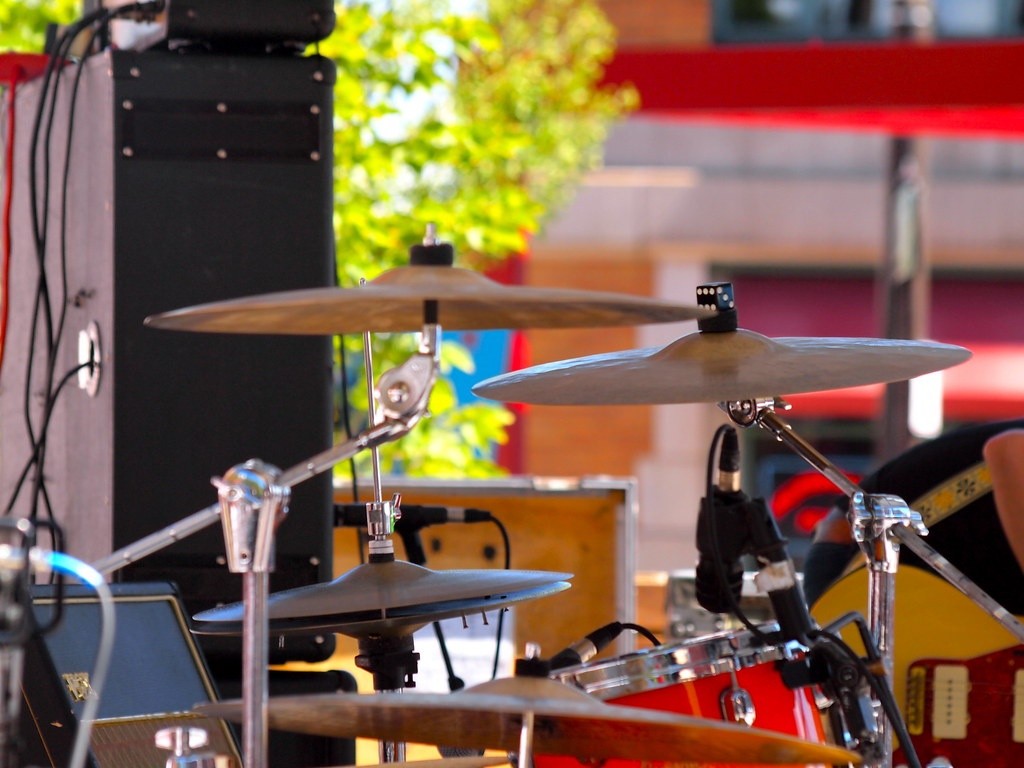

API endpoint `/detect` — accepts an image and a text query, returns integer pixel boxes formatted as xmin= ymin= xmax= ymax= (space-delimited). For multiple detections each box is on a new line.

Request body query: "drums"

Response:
xmin=510 ymin=619 xmax=833 ymax=768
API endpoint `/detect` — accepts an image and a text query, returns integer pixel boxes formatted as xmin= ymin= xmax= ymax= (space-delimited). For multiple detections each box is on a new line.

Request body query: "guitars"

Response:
xmin=805 ymin=557 xmax=1024 ymax=768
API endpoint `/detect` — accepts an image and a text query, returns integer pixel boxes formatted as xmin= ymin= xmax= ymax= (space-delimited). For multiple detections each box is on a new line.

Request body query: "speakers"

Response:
xmin=0 ymin=52 xmax=335 ymax=667
xmin=0 ymin=581 xmax=244 ymax=768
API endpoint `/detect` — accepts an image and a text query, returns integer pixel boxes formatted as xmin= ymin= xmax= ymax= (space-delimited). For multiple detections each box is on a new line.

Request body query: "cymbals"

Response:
xmin=142 ymin=264 xmax=721 ymax=337
xmin=470 ymin=329 xmax=975 ymax=407
xmin=188 ymin=560 xmax=577 ymax=655
xmin=190 ymin=676 xmax=866 ymax=764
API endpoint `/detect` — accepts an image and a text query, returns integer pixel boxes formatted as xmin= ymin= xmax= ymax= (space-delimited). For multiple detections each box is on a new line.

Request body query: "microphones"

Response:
xmin=693 ymin=427 xmax=747 ymax=615
xmin=334 ymin=504 xmax=493 ymax=525
xmin=545 ymin=621 xmax=623 ymax=673
